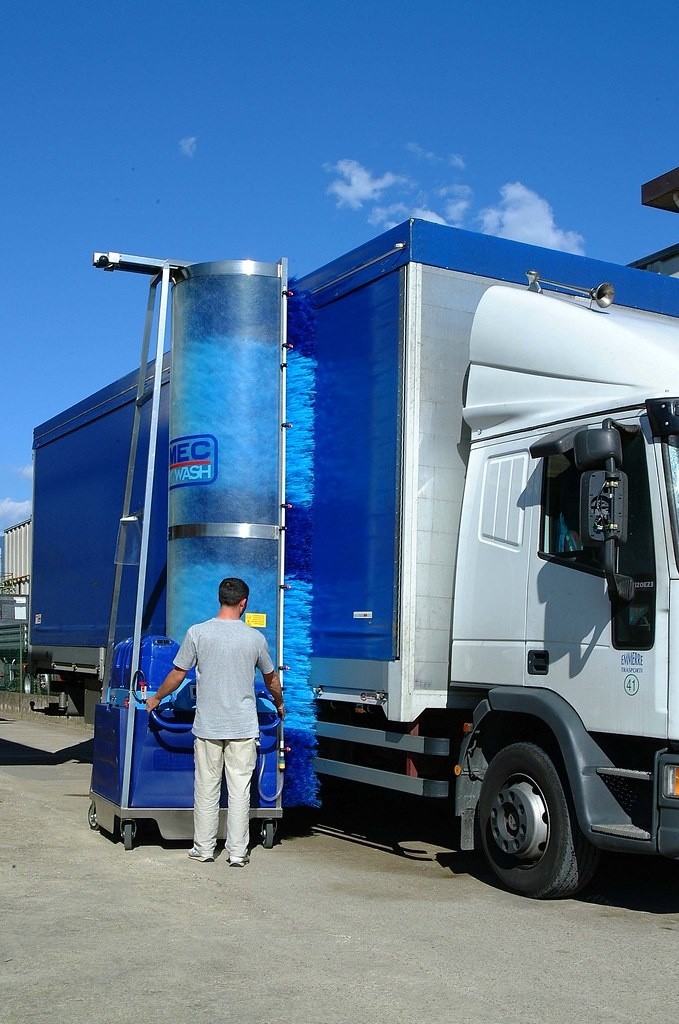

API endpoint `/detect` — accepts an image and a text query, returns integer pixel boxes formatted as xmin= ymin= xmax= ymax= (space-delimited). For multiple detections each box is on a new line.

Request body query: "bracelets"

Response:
xmin=278 ymin=704 xmax=284 ymax=711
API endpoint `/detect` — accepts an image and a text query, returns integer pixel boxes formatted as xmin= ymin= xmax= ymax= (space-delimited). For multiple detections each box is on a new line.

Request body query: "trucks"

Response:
xmin=24 ymin=215 xmax=679 ymax=902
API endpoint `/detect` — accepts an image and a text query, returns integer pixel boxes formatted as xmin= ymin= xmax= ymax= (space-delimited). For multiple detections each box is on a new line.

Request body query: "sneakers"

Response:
xmin=188 ymin=847 xmax=214 ymax=862
xmin=229 ymin=855 xmax=250 ymax=867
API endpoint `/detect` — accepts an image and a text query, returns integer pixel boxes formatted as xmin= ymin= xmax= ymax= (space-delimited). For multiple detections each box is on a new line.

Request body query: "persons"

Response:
xmin=146 ymin=578 xmax=286 ymax=866
